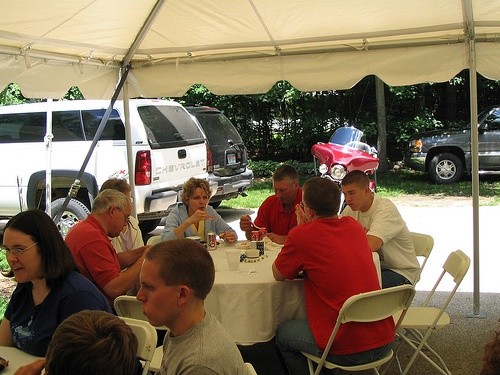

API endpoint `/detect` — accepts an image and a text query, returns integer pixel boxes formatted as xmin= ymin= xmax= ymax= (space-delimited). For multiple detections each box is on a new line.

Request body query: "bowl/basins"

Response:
xmin=236 ymin=242 xmax=249 ymax=249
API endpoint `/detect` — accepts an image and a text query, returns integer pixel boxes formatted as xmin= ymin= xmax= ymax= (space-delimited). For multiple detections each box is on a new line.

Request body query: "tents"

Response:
xmin=0 ymin=0 xmax=500 ymax=314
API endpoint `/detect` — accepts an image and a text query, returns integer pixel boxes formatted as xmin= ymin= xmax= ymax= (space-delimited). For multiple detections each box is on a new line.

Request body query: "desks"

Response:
xmin=203 ymin=240 xmax=382 ymax=374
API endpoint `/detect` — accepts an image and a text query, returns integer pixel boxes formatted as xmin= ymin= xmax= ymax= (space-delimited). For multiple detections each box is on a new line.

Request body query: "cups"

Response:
xmin=245 ymin=250 xmax=260 ymax=273
xmin=250 ymin=240 xmax=266 ymax=259
xmin=187 ymin=236 xmax=201 ymax=243
xmin=225 ymin=250 xmax=240 ymax=271
xmin=206 ymin=233 xmax=217 ymax=250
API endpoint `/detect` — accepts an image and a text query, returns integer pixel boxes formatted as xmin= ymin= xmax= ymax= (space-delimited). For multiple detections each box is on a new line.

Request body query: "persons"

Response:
xmin=63 ymin=175 xmax=155 ymax=315
xmin=159 ymin=177 xmax=237 ymax=245
xmin=339 ymin=169 xmax=421 ymax=287
xmin=478 ymin=318 xmax=500 ymax=375
xmin=272 ymin=176 xmax=398 ymax=375
xmin=239 ymin=164 xmax=302 ymax=247
xmin=0 ymin=205 xmax=247 ymax=375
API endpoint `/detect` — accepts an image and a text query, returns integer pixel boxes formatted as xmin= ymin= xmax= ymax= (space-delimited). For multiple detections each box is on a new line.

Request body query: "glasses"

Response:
xmin=109 ymin=205 xmax=130 ymax=222
xmin=0 ymin=242 xmax=39 ymax=257
xmin=129 ymin=196 xmax=134 ymax=203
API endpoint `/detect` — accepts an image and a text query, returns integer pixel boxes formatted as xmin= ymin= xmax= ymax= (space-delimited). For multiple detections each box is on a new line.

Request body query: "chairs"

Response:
xmin=382 ymin=250 xmax=471 ymax=375
xmin=111 ymin=316 xmax=158 ymax=375
xmin=400 ymin=232 xmax=434 ymax=288
xmin=114 ymin=295 xmax=170 ymax=375
xmin=300 ymin=284 xmax=417 ymax=375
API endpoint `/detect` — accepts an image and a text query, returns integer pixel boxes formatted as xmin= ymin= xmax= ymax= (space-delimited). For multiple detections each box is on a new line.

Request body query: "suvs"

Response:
xmin=182 ymin=105 xmax=254 ymax=208
xmin=0 ymin=94 xmax=218 ymax=243
xmin=405 ymin=104 xmax=500 ymax=184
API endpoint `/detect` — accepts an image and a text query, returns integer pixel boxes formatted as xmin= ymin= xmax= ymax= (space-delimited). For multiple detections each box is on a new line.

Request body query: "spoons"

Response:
xmin=242 ymin=215 xmax=266 ymax=230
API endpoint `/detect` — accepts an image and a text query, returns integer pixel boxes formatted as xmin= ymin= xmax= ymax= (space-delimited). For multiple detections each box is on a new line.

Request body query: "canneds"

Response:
xmin=207 ymin=232 xmax=216 ymax=251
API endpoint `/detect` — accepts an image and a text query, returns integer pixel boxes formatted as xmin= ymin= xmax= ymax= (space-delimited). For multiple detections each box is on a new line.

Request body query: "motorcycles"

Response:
xmin=310 ymin=126 xmax=380 ymax=218
